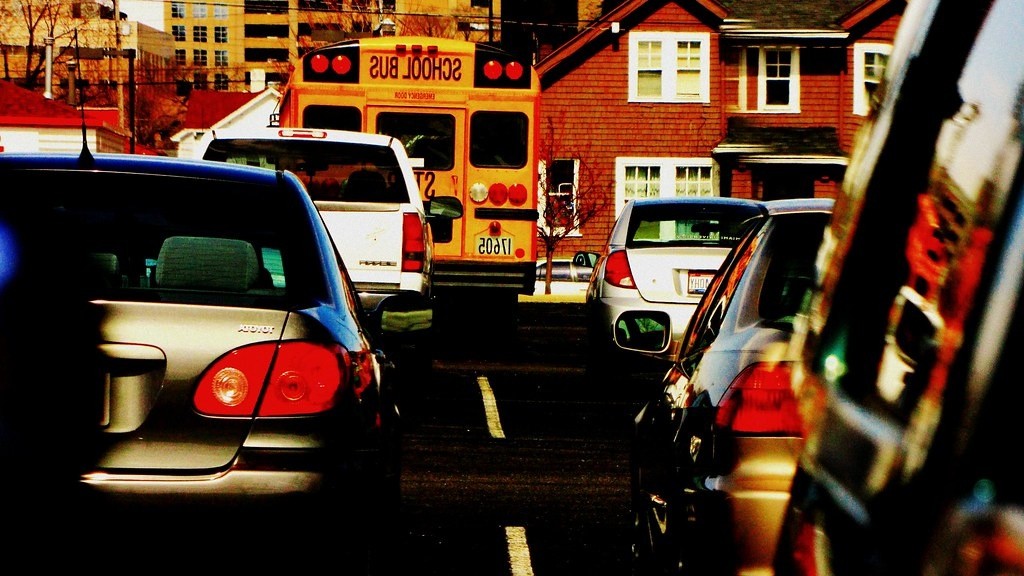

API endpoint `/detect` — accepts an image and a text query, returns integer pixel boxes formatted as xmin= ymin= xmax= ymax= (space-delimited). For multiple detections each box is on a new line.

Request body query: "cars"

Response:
xmin=573 ymin=194 xmax=769 ymax=376
xmin=531 ymin=257 xmax=597 ymax=294
xmin=628 ymin=197 xmax=838 ymax=576
xmin=0 ymin=150 xmax=438 ymax=551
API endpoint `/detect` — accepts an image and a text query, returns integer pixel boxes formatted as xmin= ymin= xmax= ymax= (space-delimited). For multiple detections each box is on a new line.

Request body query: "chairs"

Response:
xmin=347 ymin=170 xmax=387 ymax=204
xmin=154 ymin=234 xmax=258 ymax=290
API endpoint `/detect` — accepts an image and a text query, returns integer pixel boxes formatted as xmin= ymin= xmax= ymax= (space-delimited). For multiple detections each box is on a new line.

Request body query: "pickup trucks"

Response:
xmin=175 ymin=123 xmax=463 ymax=379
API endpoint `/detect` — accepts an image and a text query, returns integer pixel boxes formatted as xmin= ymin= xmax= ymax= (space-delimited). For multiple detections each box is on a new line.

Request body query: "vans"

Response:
xmin=748 ymin=1 xmax=1024 ymax=576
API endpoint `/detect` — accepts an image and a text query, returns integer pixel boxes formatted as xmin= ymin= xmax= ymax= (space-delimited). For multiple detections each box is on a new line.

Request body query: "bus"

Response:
xmin=268 ymin=17 xmax=543 ymax=338
